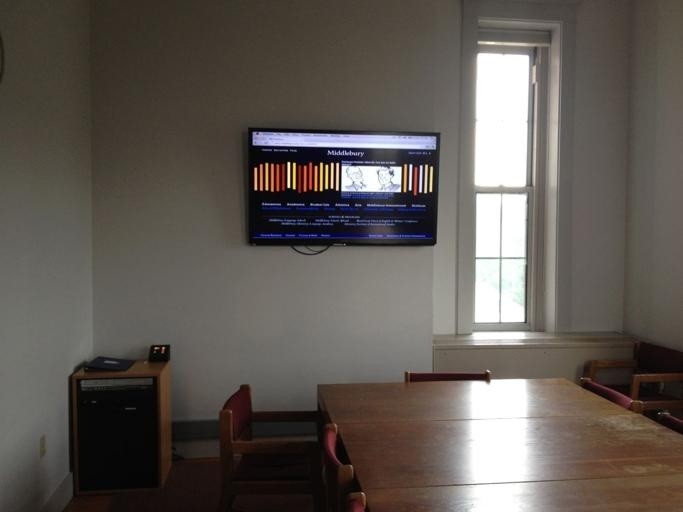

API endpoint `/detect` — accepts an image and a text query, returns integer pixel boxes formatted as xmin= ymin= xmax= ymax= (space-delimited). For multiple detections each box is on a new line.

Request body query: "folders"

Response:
xmin=85 ymin=357 xmax=135 ymax=371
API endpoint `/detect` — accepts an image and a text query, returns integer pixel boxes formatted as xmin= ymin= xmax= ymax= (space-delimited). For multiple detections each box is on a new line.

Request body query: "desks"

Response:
xmin=69 ymin=358 xmax=174 ymax=496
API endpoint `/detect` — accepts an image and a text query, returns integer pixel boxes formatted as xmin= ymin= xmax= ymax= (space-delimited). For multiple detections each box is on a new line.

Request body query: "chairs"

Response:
xmin=581 ymin=339 xmax=682 ymax=415
xmin=215 ymin=382 xmax=368 ymax=512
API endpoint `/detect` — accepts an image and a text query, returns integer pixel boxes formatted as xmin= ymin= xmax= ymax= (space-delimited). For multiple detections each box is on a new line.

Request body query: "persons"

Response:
xmin=344 ymin=166 xmax=367 ymax=192
xmin=375 ymin=165 xmax=401 ymax=192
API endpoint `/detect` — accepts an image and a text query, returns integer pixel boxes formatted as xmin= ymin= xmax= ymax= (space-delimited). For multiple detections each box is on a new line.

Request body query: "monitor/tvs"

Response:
xmin=248 ymin=127 xmax=441 ymax=245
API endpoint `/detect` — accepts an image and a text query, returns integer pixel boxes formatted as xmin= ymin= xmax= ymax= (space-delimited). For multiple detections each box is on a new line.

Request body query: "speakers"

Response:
xmin=77 ymin=377 xmax=159 ymax=491
